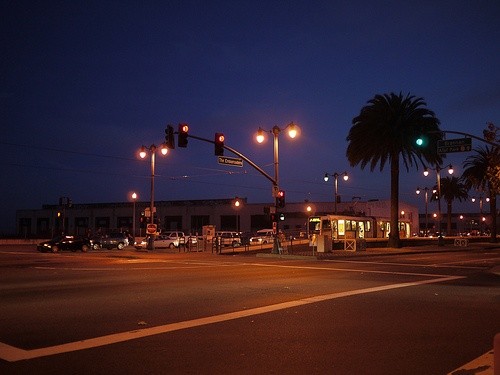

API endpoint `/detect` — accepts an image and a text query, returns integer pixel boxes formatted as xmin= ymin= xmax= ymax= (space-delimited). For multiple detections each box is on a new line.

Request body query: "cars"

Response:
xmin=37 ymin=235 xmax=93 ymax=253
xmin=134 ymin=235 xmax=179 ymax=250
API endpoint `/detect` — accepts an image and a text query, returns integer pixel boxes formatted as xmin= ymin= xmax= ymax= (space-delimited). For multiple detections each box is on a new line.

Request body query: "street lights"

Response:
xmin=416 ymin=184 xmax=436 ymax=237
xmin=132 ymin=192 xmax=137 ymax=238
xmin=423 ymin=163 xmax=454 ymax=240
xmin=139 ymin=140 xmax=169 ymax=250
xmin=256 ymin=121 xmax=297 ymax=254
xmin=234 ymin=201 xmax=240 ymax=232
xmin=471 ymin=193 xmax=490 ymax=236
xmin=324 ymin=172 xmax=348 ymax=215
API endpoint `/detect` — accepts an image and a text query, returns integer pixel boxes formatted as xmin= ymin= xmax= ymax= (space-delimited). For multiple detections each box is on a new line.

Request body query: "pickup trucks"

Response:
xmin=161 ymin=231 xmax=198 ymax=249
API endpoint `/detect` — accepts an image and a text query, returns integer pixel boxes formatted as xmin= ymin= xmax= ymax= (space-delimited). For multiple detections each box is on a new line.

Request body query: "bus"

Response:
xmin=304 ymin=214 xmax=411 ymax=247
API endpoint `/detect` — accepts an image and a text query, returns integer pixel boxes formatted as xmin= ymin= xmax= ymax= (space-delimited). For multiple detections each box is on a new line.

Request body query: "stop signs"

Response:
xmin=146 ymin=224 xmax=157 ymax=235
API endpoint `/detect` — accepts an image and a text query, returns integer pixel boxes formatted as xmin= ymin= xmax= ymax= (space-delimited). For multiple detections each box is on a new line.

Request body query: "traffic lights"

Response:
xmin=165 ymin=124 xmax=175 ymax=149
xmin=278 ymin=191 xmax=285 ymax=208
xmin=178 ymin=121 xmax=190 ymax=148
xmin=215 ymin=132 xmax=225 ymax=153
xmin=415 ymin=138 xmax=424 ymax=147
xmin=271 ymin=214 xmax=277 ymax=221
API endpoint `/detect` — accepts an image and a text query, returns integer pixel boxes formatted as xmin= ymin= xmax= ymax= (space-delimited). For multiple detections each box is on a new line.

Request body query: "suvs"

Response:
xmin=214 ymin=231 xmax=241 ymax=247
xmin=249 ymin=229 xmax=282 ymax=246
xmin=89 ymin=231 xmax=130 ymax=250
